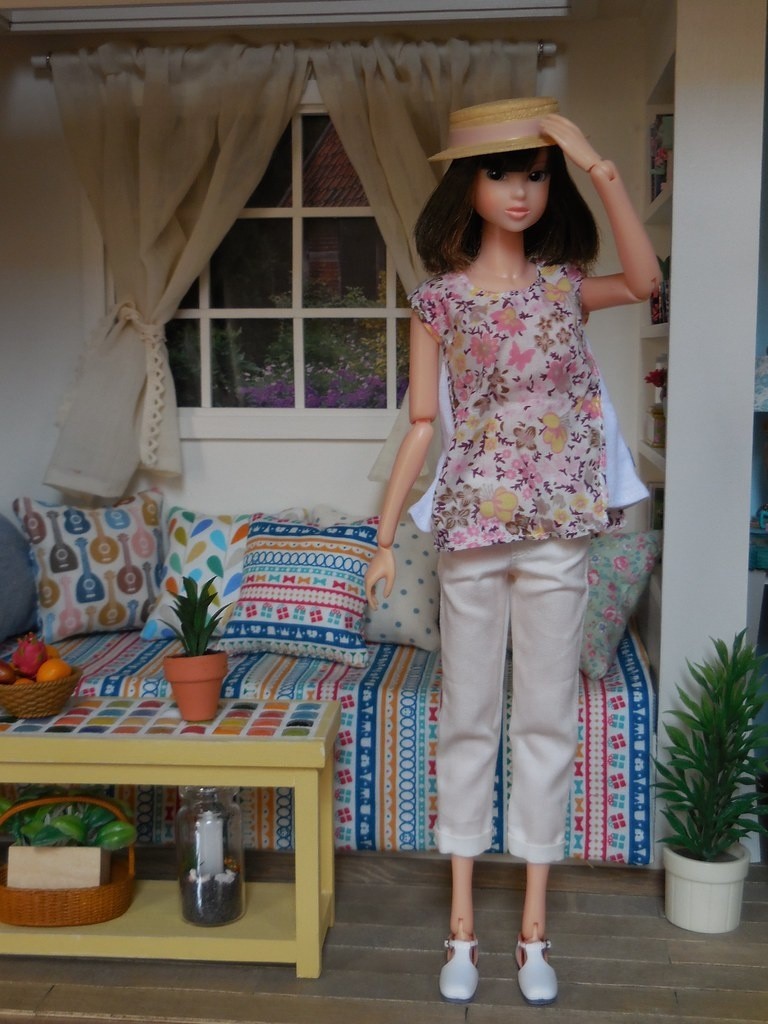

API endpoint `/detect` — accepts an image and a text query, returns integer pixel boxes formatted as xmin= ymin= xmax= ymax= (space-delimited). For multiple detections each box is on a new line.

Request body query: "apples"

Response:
xmin=0 ymin=658 xmax=16 ymax=684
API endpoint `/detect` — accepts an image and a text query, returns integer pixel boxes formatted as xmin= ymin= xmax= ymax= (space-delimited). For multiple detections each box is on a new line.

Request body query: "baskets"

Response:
xmin=0 ymin=794 xmax=137 ymax=928
xmin=0 ymin=663 xmax=81 ymax=720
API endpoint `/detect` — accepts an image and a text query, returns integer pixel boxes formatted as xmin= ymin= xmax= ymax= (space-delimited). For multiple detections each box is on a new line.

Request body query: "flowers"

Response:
xmin=642 ymin=366 xmax=668 ymax=395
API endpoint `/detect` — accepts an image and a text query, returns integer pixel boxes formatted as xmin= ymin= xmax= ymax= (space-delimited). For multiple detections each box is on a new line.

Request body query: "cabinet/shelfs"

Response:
xmin=634 ymin=47 xmax=677 ymax=656
xmin=0 ymin=695 xmax=345 ymax=981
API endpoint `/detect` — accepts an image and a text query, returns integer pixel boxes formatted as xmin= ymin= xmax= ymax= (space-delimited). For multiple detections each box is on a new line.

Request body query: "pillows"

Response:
xmin=0 ymin=512 xmax=38 ymax=642
xmin=12 ymin=488 xmax=167 ymax=644
xmin=310 ymin=504 xmax=441 ymax=651
xmin=218 ymin=513 xmax=380 ymax=668
xmin=579 ymin=529 xmax=662 ymax=682
xmin=137 ymin=506 xmax=306 ymax=642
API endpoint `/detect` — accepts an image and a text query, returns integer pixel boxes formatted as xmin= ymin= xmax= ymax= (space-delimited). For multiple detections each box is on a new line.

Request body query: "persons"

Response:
xmin=364 ymin=97 xmax=662 ymax=1004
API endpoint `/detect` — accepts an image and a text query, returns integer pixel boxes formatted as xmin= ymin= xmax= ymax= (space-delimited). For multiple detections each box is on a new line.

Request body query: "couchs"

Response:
xmin=0 ymin=632 xmax=653 ymax=870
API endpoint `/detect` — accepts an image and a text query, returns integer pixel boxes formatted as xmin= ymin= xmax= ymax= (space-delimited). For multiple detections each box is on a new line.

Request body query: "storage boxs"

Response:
xmin=643 ymin=409 xmax=665 ymax=447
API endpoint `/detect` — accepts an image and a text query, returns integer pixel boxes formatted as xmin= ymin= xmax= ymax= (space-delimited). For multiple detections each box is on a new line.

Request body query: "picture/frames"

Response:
xmin=647 ymin=482 xmax=664 ymax=529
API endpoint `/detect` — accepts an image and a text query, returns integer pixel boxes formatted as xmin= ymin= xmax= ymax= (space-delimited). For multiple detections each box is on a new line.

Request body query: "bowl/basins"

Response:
xmin=1 ymin=666 xmax=82 ymax=718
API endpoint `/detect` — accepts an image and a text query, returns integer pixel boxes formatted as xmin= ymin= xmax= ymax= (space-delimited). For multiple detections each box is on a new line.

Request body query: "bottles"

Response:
xmin=176 ymin=785 xmax=246 ymax=927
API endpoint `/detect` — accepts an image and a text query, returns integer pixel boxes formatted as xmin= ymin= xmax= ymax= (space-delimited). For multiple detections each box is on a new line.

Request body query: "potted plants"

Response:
xmin=648 ymin=625 xmax=768 ymax=933
xmin=0 ymin=785 xmax=137 ymax=890
xmin=650 ymin=255 xmax=671 ymax=323
xmin=157 ymin=577 xmax=232 ymax=722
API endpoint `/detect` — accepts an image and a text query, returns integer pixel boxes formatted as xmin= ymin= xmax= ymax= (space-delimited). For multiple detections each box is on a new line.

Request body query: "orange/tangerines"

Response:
xmin=9 ymin=645 xmax=71 ymax=685
